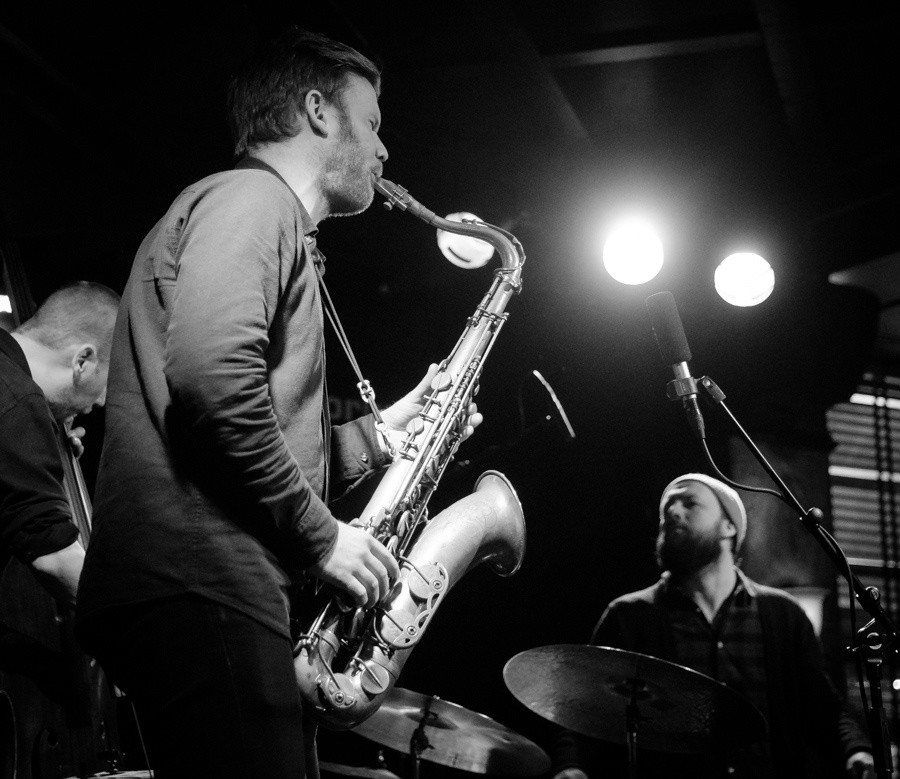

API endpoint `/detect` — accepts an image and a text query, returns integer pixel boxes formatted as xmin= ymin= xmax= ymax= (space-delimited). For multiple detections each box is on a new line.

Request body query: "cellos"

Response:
xmin=0 ymin=229 xmax=151 ymax=779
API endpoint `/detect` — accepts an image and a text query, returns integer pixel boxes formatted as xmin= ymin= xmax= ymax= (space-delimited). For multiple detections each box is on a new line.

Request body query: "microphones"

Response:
xmin=644 ymin=292 xmax=707 ymax=440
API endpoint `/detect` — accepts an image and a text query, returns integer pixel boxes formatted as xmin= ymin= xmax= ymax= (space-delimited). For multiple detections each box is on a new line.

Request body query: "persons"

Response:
xmin=75 ymin=30 xmax=483 ymax=779
xmin=535 ymin=473 xmax=874 ymax=778
xmin=0 ymin=280 xmax=123 ymax=779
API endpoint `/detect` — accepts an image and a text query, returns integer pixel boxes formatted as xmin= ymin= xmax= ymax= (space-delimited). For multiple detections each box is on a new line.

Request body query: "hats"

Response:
xmin=663 ymin=473 xmax=748 ymax=553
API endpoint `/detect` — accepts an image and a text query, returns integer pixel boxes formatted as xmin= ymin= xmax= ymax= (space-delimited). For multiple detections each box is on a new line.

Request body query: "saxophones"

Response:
xmin=293 ymin=176 xmax=527 ymax=729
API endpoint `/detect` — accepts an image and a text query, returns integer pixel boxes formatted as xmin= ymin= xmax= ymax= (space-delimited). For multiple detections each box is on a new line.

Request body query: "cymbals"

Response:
xmin=349 ymin=686 xmax=550 ymax=776
xmin=503 ymin=643 xmax=769 ymax=756
